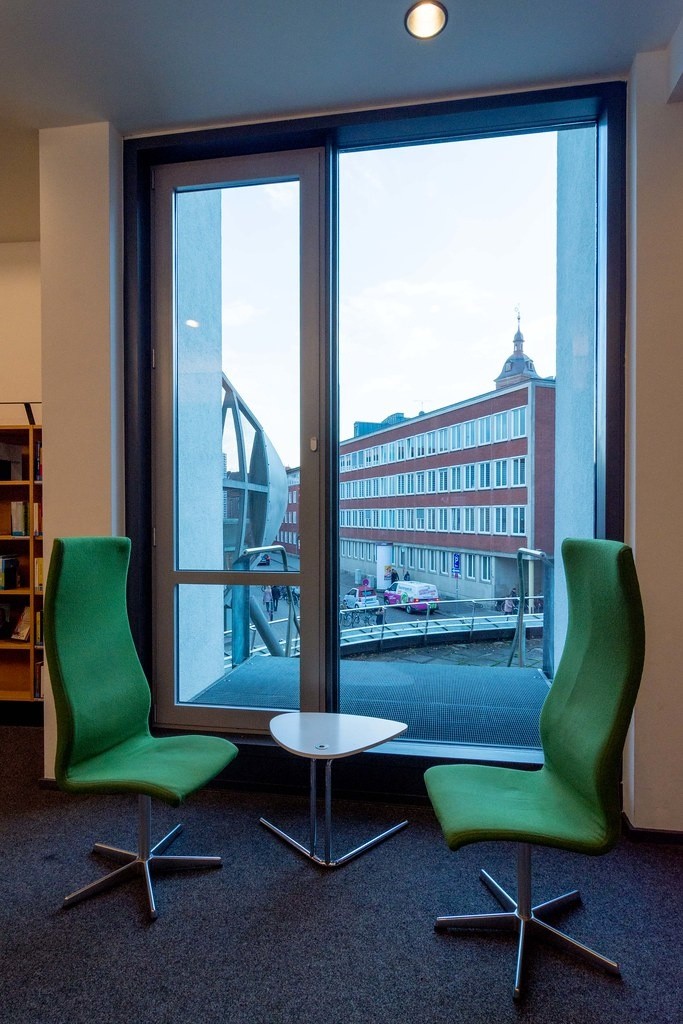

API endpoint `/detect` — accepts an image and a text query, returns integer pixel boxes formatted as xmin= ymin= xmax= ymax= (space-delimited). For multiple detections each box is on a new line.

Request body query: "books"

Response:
xmin=0 ymin=552 xmax=43 ymax=591
xmin=0 ymin=440 xmax=42 ymax=481
xmin=0 ymin=600 xmax=43 ymax=646
xmin=0 ymin=497 xmax=43 ymax=536
xmin=32 ymin=659 xmax=44 ymax=700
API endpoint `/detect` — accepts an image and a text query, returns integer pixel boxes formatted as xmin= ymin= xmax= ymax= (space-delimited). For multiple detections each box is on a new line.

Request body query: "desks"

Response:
xmin=258 ymin=711 xmax=410 ymax=870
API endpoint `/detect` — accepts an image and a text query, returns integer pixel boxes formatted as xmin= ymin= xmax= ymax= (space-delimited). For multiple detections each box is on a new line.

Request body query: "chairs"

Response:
xmin=423 ymin=536 xmax=645 ymax=1002
xmin=43 ymin=536 xmax=240 ymax=921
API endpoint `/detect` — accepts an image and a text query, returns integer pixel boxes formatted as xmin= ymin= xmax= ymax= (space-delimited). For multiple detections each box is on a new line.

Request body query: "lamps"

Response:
xmin=403 ymin=0 xmax=448 ymax=41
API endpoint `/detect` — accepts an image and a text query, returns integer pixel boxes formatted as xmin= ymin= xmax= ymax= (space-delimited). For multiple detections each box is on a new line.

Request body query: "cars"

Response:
xmin=343 ymin=587 xmax=379 ymax=613
xmin=258 ymin=554 xmax=270 ymax=566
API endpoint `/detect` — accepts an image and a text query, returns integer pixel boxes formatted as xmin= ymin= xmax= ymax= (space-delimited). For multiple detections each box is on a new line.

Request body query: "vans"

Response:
xmin=383 ymin=581 xmax=439 ymax=614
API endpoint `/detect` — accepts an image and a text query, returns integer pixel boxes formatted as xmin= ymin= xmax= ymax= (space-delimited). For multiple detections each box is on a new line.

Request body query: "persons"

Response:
xmin=391 ymin=569 xmax=411 ymax=584
xmin=0 ymin=608 xmax=10 ymax=638
xmin=340 ymin=599 xmax=347 ymax=621
xmin=504 ymin=588 xmax=529 ymax=616
xmin=375 ymin=608 xmax=385 ymax=625
xmin=263 ymin=586 xmax=281 ymax=612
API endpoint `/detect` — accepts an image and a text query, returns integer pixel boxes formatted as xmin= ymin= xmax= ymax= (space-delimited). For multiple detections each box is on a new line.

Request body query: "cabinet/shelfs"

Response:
xmin=0 ymin=400 xmax=44 ymax=704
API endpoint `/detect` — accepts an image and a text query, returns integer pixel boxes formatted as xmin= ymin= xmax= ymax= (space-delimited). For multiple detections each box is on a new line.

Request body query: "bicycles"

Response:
xmin=496 ymin=594 xmax=543 ymax=615
xmin=339 ymin=603 xmax=375 ymax=627
xmin=262 ymin=585 xmax=300 ymax=608
